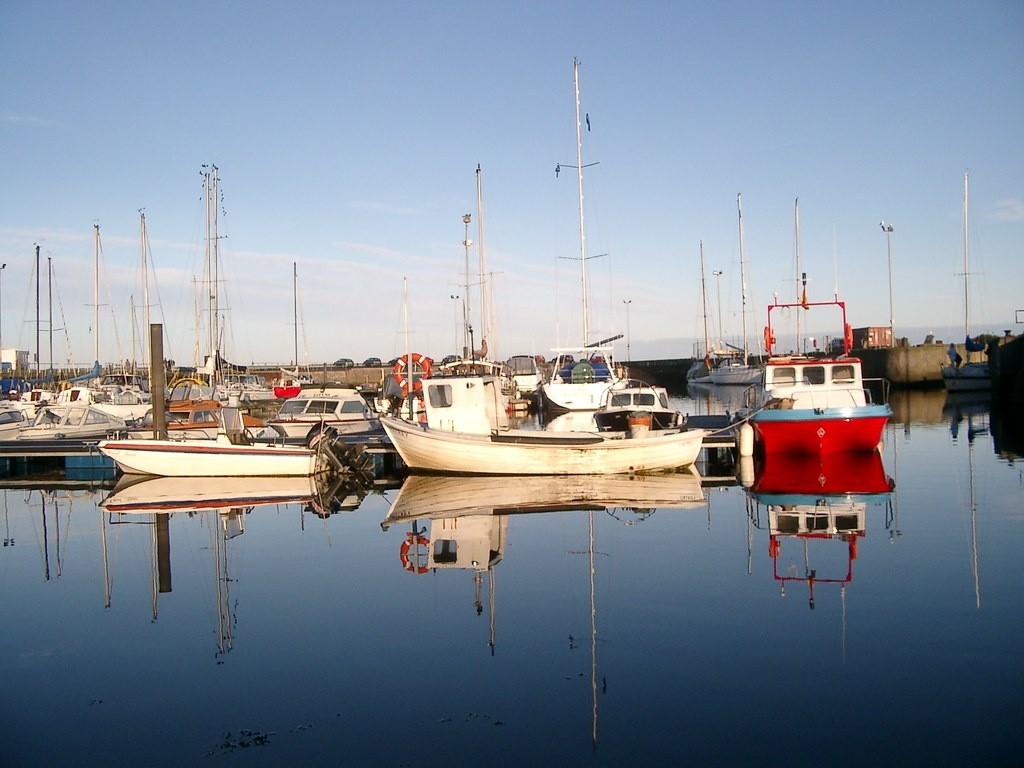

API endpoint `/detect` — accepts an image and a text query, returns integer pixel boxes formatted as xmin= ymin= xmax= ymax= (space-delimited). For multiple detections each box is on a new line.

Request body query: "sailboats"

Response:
xmin=0 ymin=53 xmax=1022 ymax=473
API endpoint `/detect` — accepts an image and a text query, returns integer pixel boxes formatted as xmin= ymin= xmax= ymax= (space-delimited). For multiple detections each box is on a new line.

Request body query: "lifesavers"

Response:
xmin=851 ymin=538 xmax=856 ymax=559
xmin=393 ymin=352 xmax=433 ymax=389
xmin=397 ymin=538 xmax=435 ymax=576
xmin=847 ymin=323 xmax=854 ymax=351
xmin=763 ymin=326 xmax=771 ymax=349
xmin=769 ymin=539 xmax=775 ymax=558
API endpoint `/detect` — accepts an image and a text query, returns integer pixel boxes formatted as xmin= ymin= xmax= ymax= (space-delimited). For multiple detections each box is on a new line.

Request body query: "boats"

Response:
xmin=95 ymin=472 xmax=374 ymax=517
xmin=733 ymin=272 xmax=896 ymax=495
xmin=378 ymin=470 xmax=708 ymax=575
xmin=375 ymin=378 xmax=705 ymax=473
xmin=93 ymin=424 xmax=366 ymax=477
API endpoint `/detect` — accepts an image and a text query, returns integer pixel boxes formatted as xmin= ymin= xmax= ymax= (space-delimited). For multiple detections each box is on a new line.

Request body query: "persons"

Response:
xmin=704 ymin=353 xmax=712 ymax=372
xmin=948 ymin=343 xmax=962 ymax=368
